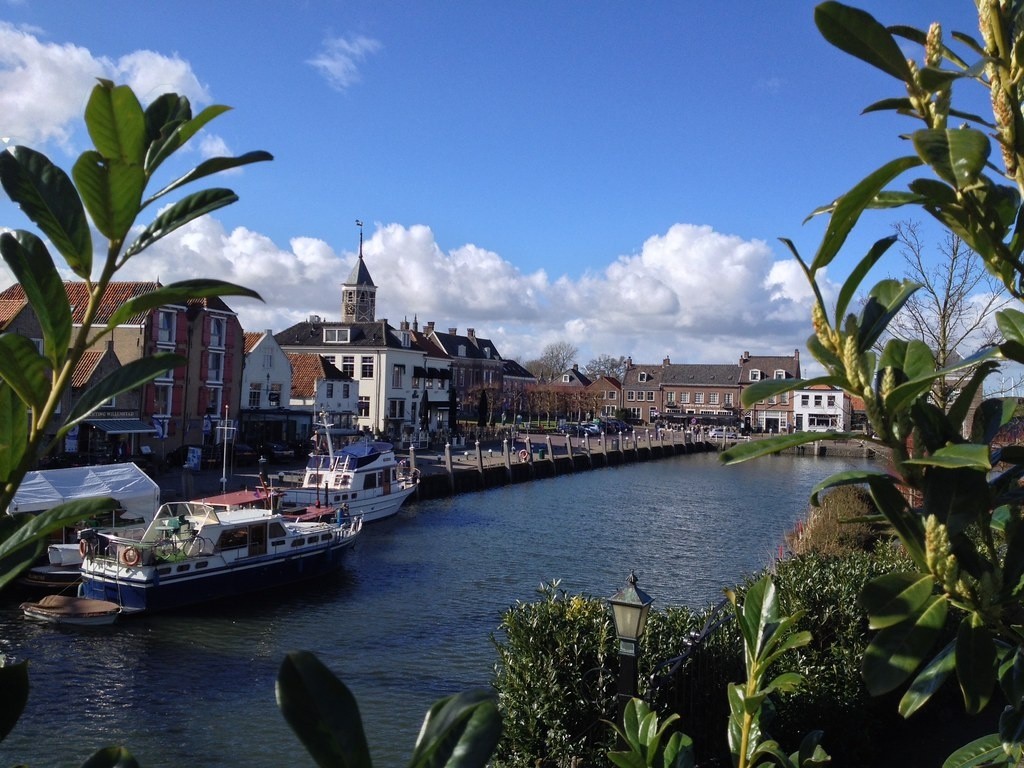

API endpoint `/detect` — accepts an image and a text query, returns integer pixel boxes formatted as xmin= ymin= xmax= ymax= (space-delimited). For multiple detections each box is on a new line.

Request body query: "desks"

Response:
xmin=155 ymin=526 xmax=175 ymax=541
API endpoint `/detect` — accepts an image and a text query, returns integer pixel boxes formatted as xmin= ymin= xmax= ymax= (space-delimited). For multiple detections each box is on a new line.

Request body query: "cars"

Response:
xmin=166 ymin=444 xmax=209 ymax=468
xmin=213 ymin=440 xmax=257 ymax=466
xmin=557 ymin=418 xmax=633 ymax=437
xmin=709 ymin=428 xmax=737 ymax=439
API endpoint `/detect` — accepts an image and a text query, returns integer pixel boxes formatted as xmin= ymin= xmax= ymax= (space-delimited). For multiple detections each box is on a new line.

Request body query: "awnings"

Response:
xmin=87 ymin=420 xmax=157 ymax=433
xmin=670 ymin=414 xmax=736 ymax=419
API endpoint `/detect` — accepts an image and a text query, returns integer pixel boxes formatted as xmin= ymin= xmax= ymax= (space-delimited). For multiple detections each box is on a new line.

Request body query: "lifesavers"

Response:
xmin=80 ymin=538 xmax=89 ymax=558
xmin=121 ymin=547 xmax=140 ymax=566
xmin=519 ymin=449 xmax=528 ymax=460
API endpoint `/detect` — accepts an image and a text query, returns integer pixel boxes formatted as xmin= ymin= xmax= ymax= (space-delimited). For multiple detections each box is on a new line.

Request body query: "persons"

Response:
xmin=502 ymin=411 xmax=506 ymax=426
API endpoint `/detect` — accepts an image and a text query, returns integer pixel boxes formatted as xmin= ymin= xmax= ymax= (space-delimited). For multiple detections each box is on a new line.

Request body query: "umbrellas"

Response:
xmin=419 ymin=390 xmax=428 ymax=429
xmin=478 ymin=391 xmax=488 ymax=437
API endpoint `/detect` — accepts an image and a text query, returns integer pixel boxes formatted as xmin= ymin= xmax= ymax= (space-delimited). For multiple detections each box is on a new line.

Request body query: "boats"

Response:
xmin=281 ymin=406 xmax=416 ymax=527
xmin=79 ymin=502 xmax=363 ymax=614
xmin=25 ymin=544 xmax=84 ymax=589
xmin=189 ymin=473 xmax=339 ymax=523
xmin=19 ymin=594 xmax=121 ymax=626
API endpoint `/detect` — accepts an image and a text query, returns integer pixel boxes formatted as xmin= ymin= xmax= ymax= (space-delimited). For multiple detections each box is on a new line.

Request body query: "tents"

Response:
xmin=5 ymin=462 xmax=160 ymax=524
